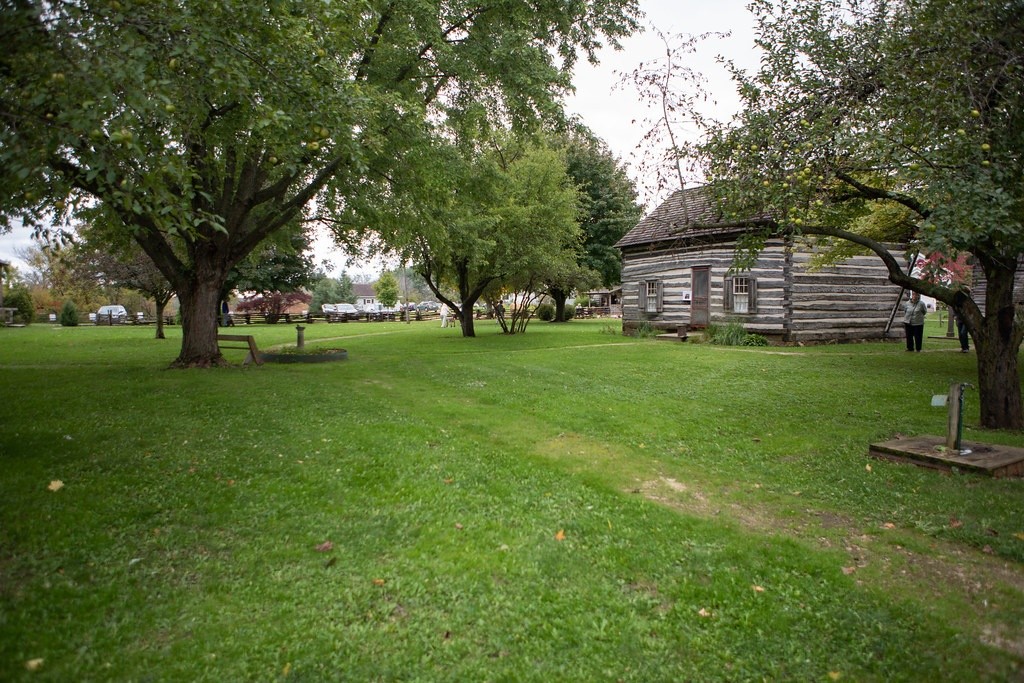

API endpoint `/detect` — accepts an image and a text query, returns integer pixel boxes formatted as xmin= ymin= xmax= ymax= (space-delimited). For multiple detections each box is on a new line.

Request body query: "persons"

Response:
xmin=222 ymin=302 xmax=229 ymax=327
xmin=902 ymin=291 xmax=927 ymax=352
xmin=953 ymin=287 xmax=970 ymax=352
xmin=440 ymin=303 xmax=451 ymax=327
xmin=495 ymin=300 xmax=506 ymax=323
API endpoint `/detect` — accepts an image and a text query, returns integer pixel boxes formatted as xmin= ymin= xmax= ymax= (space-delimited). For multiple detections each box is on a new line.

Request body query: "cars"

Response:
xmin=322 ymin=302 xmax=360 ymax=324
xmin=354 ymin=303 xmax=381 ymax=321
xmin=97 ymin=305 xmax=128 ymax=324
xmin=416 ymin=301 xmax=438 ymax=312
xmin=403 ymin=302 xmax=416 ymax=310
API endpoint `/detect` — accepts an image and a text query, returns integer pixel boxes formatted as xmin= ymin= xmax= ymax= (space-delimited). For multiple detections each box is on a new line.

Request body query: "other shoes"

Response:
xmin=960 ymin=349 xmax=968 ymax=353
xmin=917 ymin=350 xmax=920 ymax=352
xmin=905 ymin=350 xmax=914 ymax=352
